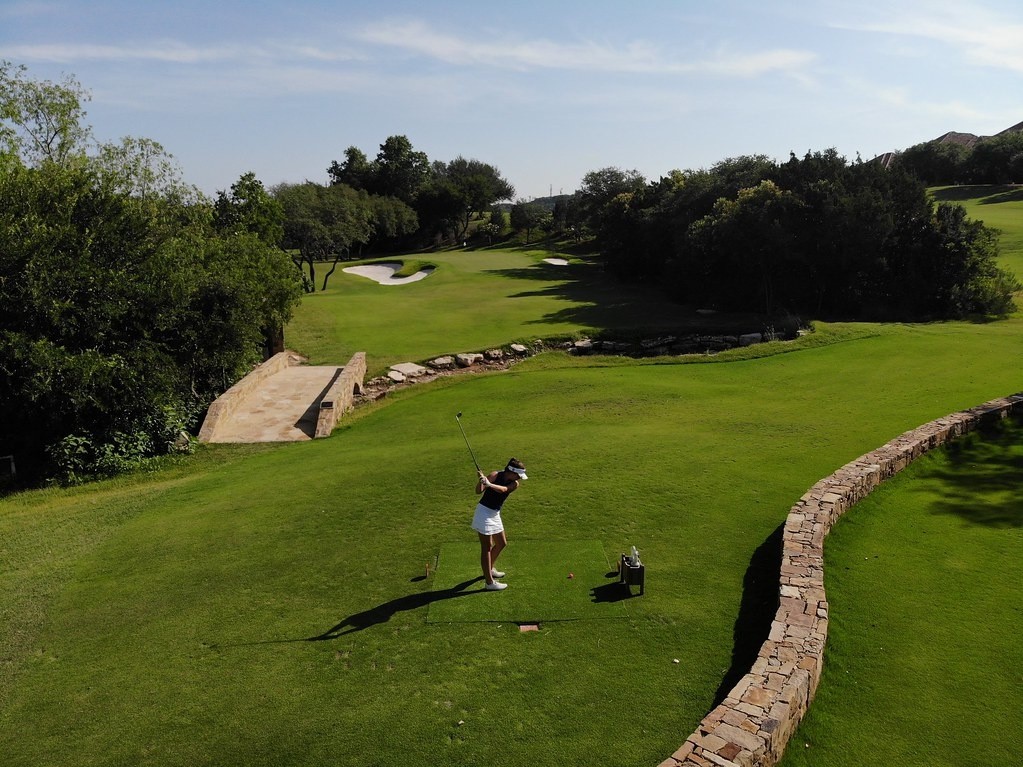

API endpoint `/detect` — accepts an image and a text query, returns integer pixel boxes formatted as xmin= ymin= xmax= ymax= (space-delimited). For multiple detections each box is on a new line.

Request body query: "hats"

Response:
xmin=508 ymin=465 xmax=528 ymax=480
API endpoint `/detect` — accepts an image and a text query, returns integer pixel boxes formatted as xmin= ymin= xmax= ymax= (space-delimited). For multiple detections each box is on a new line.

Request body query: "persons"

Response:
xmin=471 ymin=457 xmax=528 ymax=589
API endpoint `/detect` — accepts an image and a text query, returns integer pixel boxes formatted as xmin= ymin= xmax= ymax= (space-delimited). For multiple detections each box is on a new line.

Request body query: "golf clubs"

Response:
xmin=454 ymin=410 xmax=487 ymax=486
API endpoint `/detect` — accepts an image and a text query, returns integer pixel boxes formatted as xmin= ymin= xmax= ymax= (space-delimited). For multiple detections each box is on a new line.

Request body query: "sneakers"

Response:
xmin=491 ymin=568 xmax=505 ymax=578
xmin=485 ymin=580 xmax=508 ymax=590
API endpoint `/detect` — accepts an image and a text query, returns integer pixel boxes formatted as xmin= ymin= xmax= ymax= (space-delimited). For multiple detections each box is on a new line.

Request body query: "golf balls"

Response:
xmin=569 ymin=574 xmax=573 ymax=578
